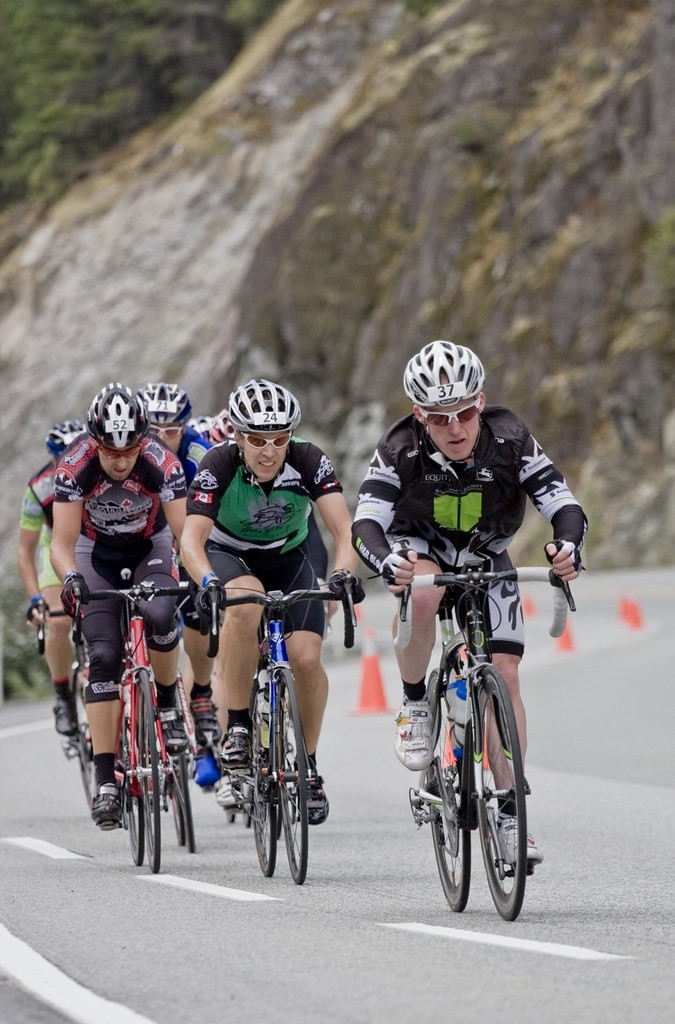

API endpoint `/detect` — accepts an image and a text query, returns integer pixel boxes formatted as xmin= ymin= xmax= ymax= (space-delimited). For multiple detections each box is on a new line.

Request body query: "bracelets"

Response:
xmin=62 ymin=571 xmax=79 ymax=581
xmin=31 ymin=595 xmax=43 ymax=604
xmin=200 ymin=572 xmax=216 ymax=588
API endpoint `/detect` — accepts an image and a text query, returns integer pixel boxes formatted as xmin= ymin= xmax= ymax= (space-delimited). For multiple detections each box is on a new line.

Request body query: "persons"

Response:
xmin=19 ymin=378 xmax=361 ymax=827
xmin=350 ymin=341 xmax=589 ymax=866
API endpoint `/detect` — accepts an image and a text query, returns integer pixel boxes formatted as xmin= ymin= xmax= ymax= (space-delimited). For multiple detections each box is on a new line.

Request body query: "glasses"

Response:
xmin=98 ymin=445 xmax=140 ymax=459
xmin=149 ymin=424 xmax=183 ymax=439
xmin=241 ymin=432 xmax=291 ymax=448
xmin=420 ymin=396 xmax=482 ymax=426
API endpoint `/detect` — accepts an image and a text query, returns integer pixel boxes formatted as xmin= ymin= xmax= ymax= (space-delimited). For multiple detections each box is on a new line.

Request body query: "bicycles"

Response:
xmin=36 ymin=582 xmax=227 ymax=875
xmin=396 ymin=567 xmax=575 ymax=922
xmin=198 ymin=572 xmax=360 ymax=885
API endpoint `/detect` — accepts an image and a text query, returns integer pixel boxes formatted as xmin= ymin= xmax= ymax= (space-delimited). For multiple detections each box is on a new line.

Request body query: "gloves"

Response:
xmin=329 ymin=569 xmax=366 ymax=605
xmin=60 ymin=571 xmax=89 ymax=620
xmin=197 ymin=573 xmax=226 ymax=620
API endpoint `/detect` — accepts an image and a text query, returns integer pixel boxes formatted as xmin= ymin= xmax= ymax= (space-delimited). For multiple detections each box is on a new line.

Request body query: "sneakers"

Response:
xmin=53 ymin=699 xmax=78 ymax=734
xmin=158 ymin=709 xmax=188 ymax=754
xmin=213 ymin=776 xmax=243 ymax=808
xmin=494 ymin=817 xmax=545 ymax=865
xmin=190 ymin=691 xmax=222 ymax=744
xmin=91 ymin=783 xmax=121 ymax=823
xmin=294 ymin=762 xmax=329 ymax=824
xmin=218 ymin=722 xmax=250 ymax=769
xmin=394 ymin=693 xmax=436 ymax=770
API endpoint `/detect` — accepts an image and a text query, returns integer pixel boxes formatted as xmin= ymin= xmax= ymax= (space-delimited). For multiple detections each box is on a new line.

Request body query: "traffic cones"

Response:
xmin=524 ymin=597 xmax=535 ymax=618
xmin=556 ymin=621 xmax=573 ymax=651
xmin=619 ymin=595 xmax=643 ymax=630
xmin=346 ymin=651 xmax=399 ymax=714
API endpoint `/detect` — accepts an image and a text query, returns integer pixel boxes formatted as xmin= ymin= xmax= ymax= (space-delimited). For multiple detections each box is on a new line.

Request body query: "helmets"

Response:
xmin=402 ymin=340 xmax=485 ymax=405
xmin=210 ymin=409 xmax=236 ymax=443
xmin=136 ymin=382 xmax=191 ymax=424
xmin=85 ymin=382 xmax=150 ymax=450
xmin=227 ymin=378 xmax=301 ymax=434
xmin=46 ymin=419 xmax=86 ymax=456
xmin=189 ymin=416 xmax=212 ymax=435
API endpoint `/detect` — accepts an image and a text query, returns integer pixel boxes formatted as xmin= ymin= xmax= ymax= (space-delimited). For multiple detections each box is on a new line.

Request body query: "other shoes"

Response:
xmin=193 ymin=746 xmax=219 ymax=786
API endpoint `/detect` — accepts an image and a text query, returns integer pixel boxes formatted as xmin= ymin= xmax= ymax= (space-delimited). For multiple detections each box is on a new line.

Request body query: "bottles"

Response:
xmin=123 ymin=680 xmax=133 ymax=715
xmin=259 ymin=691 xmax=289 ymax=748
xmin=446 ymin=678 xmax=468 ymax=757
